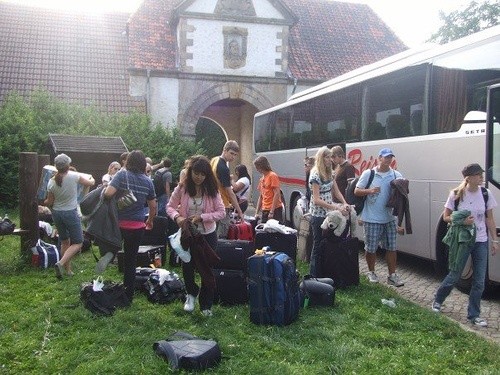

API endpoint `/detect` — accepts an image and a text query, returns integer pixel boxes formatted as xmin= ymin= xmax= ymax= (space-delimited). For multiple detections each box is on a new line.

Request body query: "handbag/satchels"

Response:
xmin=115 ymin=172 xmax=138 ymax=212
xmin=31 ymin=241 xmax=44 ymax=269
xmin=168 ymin=226 xmax=191 ymax=263
xmin=298 ymin=275 xmax=334 ymax=307
xmin=36 ymin=239 xmax=61 ymax=269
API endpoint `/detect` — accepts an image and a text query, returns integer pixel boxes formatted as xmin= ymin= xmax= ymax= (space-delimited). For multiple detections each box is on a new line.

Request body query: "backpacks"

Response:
xmin=82 ymin=278 xmax=125 ymax=316
xmin=153 ymin=169 xmax=170 ymax=196
xmin=135 ymin=268 xmax=157 ymax=289
xmin=345 ymin=169 xmax=375 ymax=216
xmin=144 ymin=269 xmax=187 ymax=305
xmin=153 ymin=333 xmax=221 ymax=375
xmin=0 ymin=217 xmax=16 ymax=235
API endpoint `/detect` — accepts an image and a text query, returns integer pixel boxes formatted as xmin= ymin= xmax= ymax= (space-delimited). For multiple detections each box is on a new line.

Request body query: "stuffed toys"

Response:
xmin=320 ymin=205 xmax=357 ymax=237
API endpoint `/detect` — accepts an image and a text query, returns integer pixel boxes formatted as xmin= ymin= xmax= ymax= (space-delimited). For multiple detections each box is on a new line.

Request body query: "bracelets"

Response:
xmin=175 ymin=214 xmax=182 ymax=221
xmin=491 ymin=240 xmax=499 ymax=244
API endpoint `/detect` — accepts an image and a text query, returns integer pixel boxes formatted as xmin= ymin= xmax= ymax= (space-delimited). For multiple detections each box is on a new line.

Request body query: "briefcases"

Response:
xmin=118 ymin=244 xmax=165 ymax=273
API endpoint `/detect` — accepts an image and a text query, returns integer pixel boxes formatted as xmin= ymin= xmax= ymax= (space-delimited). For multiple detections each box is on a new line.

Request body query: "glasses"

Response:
xmin=262 ymin=179 xmax=265 ymax=188
xmin=305 ymin=164 xmax=312 ymax=167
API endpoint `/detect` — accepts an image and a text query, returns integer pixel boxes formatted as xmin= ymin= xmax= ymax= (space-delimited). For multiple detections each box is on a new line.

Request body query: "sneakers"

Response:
xmin=467 ymin=316 xmax=487 ymax=327
xmin=184 ymin=293 xmax=196 ymax=313
xmin=366 ymin=270 xmax=379 ymax=284
xmin=388 ymin=273 xmax=404 ymax=287
xmin=431 ymin=293 xmax=442 ymax=311
xmin=95 ymin=252 xmax=114 ymax=275
xmin=64 ymin=272 xmax=75 ymax=277
xmin=199 ymin=310 xmax=212 ymax=317
xmin=53 ymin=262 xmax=63 ymax=281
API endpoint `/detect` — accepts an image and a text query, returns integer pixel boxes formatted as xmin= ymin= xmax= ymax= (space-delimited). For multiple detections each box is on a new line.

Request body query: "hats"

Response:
xmin=461 ymin=163 xmax=485 ymax=178
xmin=55 ymin=154 xmax=71 ymax=169
xmin=348 ymin=149 xmax=361 ymax=158
xmin=378 ymin=148 xmax=395 ymax=158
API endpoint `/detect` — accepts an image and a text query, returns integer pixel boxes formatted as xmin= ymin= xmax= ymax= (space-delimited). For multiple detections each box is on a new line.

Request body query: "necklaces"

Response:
xmin=193 ymin=192 xmax=203 ymax=206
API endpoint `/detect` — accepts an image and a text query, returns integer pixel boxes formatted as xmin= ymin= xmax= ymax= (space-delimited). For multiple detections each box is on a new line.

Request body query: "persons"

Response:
xmin=308 ymin=146 xmax=351 ymax=277
xmin=145 ymin=157 xmax=164 ymax=179
xmin=253 ymin=156 xmax=284 ymax=225
xmin=432 ymin=163 xmax=500 ymax=327
xmin=44 ymin=153 xmax=96 ymax=282
xmin=102 ymin=160 xmax=121 ymax=187
xmin=304 ymin=157 xmax=314 ymax=212
xmin=94 ymin=149 xmax=156 ymax=308
xmin=69 ymin=166 xmax=83 ymax=224
xmin=121 ymin=152 xmax=129 ymax=167
xmin=155 ymin=159 xmax=172 ymax=216
xmin=353 ymin=148 xmax=405 ymax=287
xmin=143 ymin=162 xmax=151 ymax=214
xmin=165 ymin=155 xmax=226 ymax=317
xmin=230 ymin=164 xmax=251 ymax=219
xmin=330 ymin=145 xmax=355 ymax=203
xmin=210 ymin=140 xmax=246 ymax=240
xmin=180 ymin=159 xmax=190 ymax=183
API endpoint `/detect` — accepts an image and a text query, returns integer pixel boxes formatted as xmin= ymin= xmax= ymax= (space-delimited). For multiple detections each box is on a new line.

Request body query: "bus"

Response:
xmin=251 ymin=25 xmax=500 ymax=295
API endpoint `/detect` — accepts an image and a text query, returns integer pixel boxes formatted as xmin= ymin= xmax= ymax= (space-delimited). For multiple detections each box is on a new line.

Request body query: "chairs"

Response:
xmin=335 ymin=129 xmax=344 ymax=142
xmin=410 ymin=110 xmax=422 ymax=135
xmin=386 ymin=115 xmax=401 ymax=138
xmin=369 ymin=122 xmax=381 ymax=141
xmin=301 ymin=131 xmax=311 ymax=147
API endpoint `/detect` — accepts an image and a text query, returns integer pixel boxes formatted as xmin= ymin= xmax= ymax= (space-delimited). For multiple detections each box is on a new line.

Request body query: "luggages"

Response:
xmin=227 ymin=220 xmax=256 ymax=245
xmin=299 ymin=214 xmax=311 ymax=264
xmin=318 ymin=208 xmax=360 ymax=288
xmin=144 ymin=199 xmax=169 ymax=243
xmin=208 ymin=239 xmax=256 ymax=305
xmin=248 ymin=245 xmax=297 ymax=325
xmin=256 ymin=222 xmax=297 ymax=258
